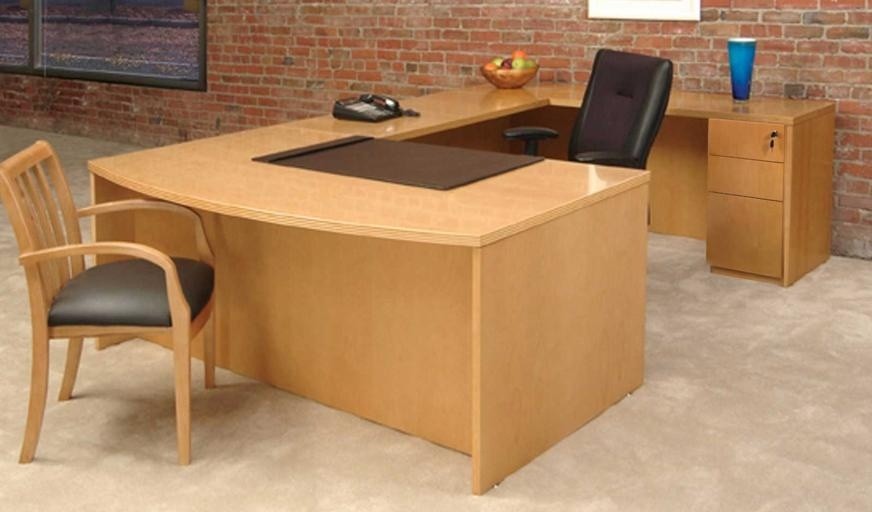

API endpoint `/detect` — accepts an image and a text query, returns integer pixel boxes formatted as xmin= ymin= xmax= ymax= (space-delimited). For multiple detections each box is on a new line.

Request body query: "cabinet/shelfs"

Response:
xmin=705 ymin=118 xmax=787 ymax=280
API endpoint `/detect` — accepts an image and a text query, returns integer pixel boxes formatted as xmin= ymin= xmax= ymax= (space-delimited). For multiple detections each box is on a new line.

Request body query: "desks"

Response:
xmin=88 ymin=123 xmax=652 ymax=495
xmin=284 ymin=85 xmax=551 ymax=145
xmin=519 ymin=83 xmax=836 ymax=285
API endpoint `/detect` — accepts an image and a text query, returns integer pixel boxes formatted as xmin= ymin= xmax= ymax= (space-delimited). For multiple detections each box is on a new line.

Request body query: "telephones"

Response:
xmin=333 ymin=93 xmax=402 ymax=122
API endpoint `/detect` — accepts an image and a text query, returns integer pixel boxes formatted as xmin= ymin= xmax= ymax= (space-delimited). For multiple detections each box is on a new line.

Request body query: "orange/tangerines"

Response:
xmin=483 ymin=63 xmax=497 ymax=71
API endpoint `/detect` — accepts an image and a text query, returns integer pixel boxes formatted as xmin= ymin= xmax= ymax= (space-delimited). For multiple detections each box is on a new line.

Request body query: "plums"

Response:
xmin=507 ymin=59 xmax=511 ymax=65
xmin=501 ymin=62 xmax=510 ymax=69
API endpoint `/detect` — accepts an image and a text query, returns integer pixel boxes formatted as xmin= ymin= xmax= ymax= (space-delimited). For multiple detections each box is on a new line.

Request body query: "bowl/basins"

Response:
xmin=479 ymin=64 xmax=541 ymax=89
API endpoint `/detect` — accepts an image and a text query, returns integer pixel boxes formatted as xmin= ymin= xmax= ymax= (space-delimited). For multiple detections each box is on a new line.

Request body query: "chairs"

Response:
xmin=1 ymin=137 xmax=216 ymax=464
xmin=502 ymin=50 xmax=672 ymax=228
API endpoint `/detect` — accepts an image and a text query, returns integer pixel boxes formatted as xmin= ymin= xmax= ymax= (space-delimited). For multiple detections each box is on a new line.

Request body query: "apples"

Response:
xmin=491 ymin=56 xmax=503 ymax=68
xmin=512 ymin=58 xmax=535 ymax=68
